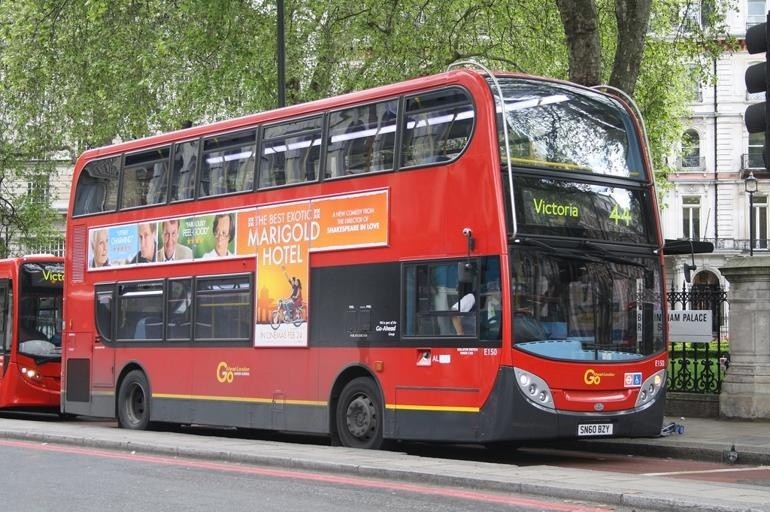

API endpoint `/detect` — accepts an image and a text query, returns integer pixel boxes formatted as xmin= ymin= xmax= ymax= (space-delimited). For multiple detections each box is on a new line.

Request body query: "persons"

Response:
xmin=88 ymin=213 xmax=235 ymax=268
xmin=451 ymin=291 xmax=476 ymax=335
xmin=281 ymin=271 xmax=303 ymax=321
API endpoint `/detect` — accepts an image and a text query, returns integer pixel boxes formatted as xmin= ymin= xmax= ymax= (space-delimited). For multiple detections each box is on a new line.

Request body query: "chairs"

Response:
xmin=96 ymin=302 xmax=110 ymax=339
xmin=141 ymin=113 xmax=454 ymax=206
xmin=135 ymin=317 xmax=160 ymax=340
xmin=434 ymin=290 xmax=459 ymax=337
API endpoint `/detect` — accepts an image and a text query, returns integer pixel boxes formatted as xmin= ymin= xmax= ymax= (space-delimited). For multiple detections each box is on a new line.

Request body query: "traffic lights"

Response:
xmin=739 ymin=8 xmax=770 ymax=171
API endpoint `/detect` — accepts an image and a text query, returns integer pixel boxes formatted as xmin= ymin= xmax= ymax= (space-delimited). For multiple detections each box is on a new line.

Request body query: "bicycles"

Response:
xmin=270 ymin=298 xmax=306 ymax=331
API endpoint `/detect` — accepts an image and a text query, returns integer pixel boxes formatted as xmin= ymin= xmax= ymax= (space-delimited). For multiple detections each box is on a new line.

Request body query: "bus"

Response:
xmin=0 ymin=252 xmax=64 ymax=408
xmin=59 ymin=53 xmax=697 ymax=450
